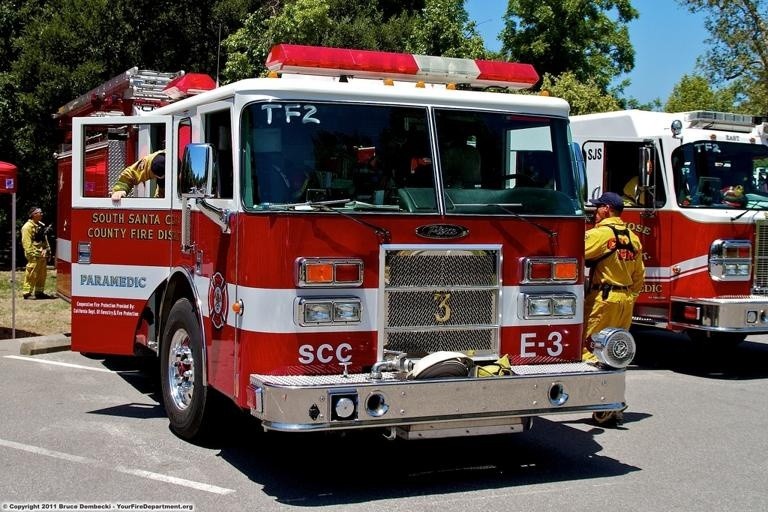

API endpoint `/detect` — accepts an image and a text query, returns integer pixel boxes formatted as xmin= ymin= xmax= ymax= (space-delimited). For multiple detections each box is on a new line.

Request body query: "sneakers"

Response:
xmin=592 ymin=403 xmax=628 ymax=426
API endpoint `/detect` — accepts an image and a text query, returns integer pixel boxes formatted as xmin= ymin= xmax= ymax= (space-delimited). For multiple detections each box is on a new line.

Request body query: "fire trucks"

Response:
xmin=52 ymin=43 xmax=637 ymax=440
xmin=465 ymin=108 xmax=768 ymax=347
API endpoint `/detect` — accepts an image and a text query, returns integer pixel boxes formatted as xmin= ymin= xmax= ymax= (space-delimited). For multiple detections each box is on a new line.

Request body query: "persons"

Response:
xmin=111 ymin=147 xmax=183 ymax=202
xmin=20 ymin=206 xmax=54 ymax=300
xmin=582 ymin=192 xmax=646 ymax=427
xmin=619 ymin=174 xmax=647 ymax=210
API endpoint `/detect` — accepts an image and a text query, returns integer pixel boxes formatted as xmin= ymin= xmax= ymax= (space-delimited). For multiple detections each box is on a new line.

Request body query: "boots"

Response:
xmin=34 ymin=293 xmax=51 ymax=299
xmin=23 ymin=294 xmax=37 ymax=300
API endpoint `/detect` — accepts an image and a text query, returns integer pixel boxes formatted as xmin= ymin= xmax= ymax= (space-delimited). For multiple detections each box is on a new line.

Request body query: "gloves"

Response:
xmin=111 ymin=190 xmax=128 ymax=202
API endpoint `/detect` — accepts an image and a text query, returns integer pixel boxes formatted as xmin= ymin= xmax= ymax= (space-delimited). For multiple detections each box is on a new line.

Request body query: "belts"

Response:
xmin=592 ymin=284 xmax=626 ymax=291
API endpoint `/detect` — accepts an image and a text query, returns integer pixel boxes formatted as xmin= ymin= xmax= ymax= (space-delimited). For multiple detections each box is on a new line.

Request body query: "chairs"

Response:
xmin=443 ymin=145 xmax=483 ymax=189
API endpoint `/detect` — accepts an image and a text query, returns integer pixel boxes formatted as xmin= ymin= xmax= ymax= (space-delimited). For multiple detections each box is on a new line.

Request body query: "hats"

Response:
xmin=588 ymin=191 xmax=624 ymax=211
